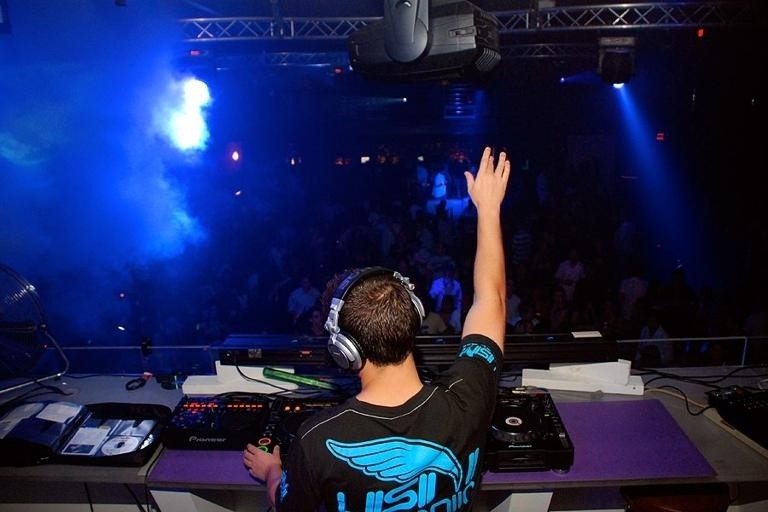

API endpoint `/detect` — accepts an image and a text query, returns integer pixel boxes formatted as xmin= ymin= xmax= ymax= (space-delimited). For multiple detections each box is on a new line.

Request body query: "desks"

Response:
xmin=0 ymin=372 xmax=167 ymax=512
xmin=146 ymin=367 xmax=768 ymax=512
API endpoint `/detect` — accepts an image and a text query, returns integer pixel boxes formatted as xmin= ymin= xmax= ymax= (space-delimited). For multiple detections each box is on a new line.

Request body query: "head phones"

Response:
xmin=323 ymin=266 xmax=426 ymax=372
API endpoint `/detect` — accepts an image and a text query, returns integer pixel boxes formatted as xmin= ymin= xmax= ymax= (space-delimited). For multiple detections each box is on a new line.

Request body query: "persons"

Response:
xmin=242 ymin=146 xmax=511 ymax=512
